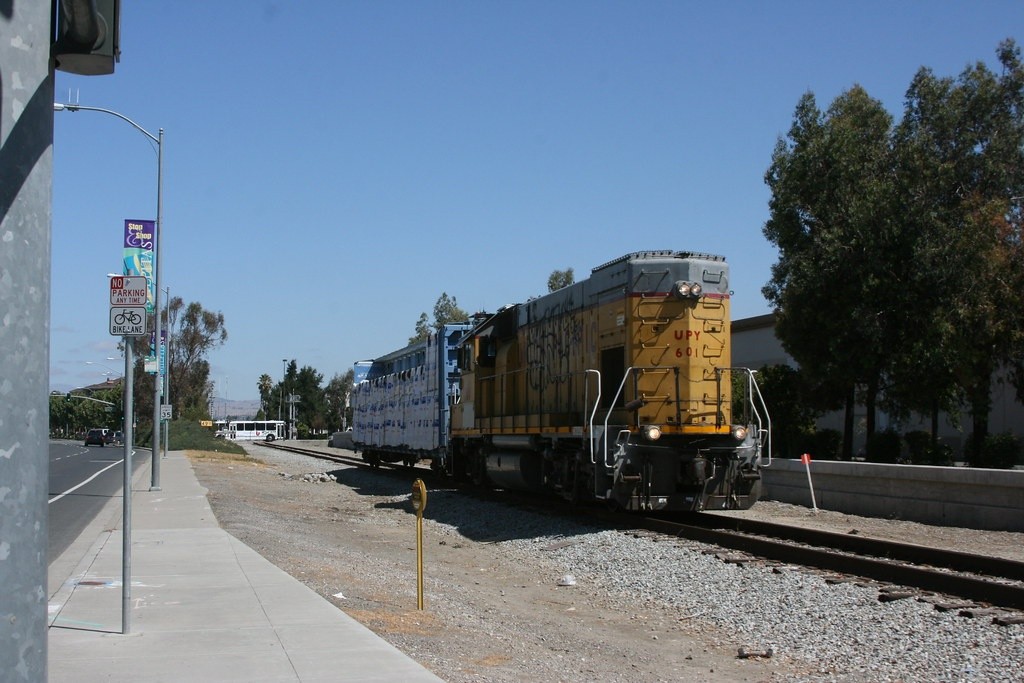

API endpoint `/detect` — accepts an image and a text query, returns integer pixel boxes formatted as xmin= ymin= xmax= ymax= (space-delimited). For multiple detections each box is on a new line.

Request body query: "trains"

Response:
xmin=351 ymin=249 xmax=773 ymax=526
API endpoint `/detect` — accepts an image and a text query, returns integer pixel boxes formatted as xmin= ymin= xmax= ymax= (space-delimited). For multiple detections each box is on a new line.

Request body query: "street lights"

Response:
xmin=54 ymin=104 xmax=168 ymax=494
xmin=283 ymin=359 xmax=287 ymax=422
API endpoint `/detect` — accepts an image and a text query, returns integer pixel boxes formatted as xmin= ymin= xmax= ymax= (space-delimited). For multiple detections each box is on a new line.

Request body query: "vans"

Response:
xmin=104 ymin=430 xmax=124 ymax=445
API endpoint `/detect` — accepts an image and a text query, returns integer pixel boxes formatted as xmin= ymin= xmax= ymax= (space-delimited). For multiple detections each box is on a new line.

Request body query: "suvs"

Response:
xmin=85 ymin=429 xmax=104 ymax=448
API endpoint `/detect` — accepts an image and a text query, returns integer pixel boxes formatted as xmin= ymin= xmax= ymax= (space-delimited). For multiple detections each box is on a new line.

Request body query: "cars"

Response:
xmin=102 ymin=428 xmax=111 ymax=436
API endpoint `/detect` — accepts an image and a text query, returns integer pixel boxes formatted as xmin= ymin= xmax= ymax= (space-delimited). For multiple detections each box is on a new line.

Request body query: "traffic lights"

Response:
xmin=66 ymin=393 xmax=71 ymax=402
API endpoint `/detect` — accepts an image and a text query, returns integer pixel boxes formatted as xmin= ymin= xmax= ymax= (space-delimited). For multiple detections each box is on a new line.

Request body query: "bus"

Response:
xmin=213 ymin=419 xmax=287 ymax=443
